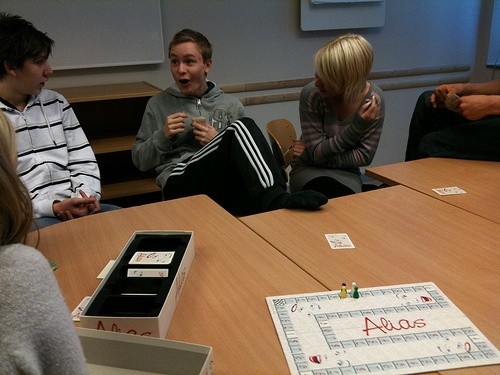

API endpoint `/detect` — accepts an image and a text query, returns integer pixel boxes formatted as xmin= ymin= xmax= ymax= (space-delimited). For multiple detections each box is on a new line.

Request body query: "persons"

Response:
xmin=132 ymin=28 xmax=329 ymax=210
xmin=0 ymin=10 xmax=123 ymax=235
xmin=0 ymin=108 xmax=89 ymax=375
xmin=404 ymin=80 xmax=500 ymax=163
xmin=290 ymin=33 xmax=385 ymax=202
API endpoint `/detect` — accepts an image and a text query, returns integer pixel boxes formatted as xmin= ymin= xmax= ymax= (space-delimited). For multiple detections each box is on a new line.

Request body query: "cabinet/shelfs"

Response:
xmin=52 ymin=82 xmax=165 ymax=200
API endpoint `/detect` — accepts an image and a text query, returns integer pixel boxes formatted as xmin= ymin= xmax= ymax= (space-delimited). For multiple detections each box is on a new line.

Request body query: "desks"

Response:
xmin=365 ymin=157 xmax=500 ymax=224
xmin=238 ymin=184 xmax=500 ymax=375
xmin=22 ymin=193 xmax=438 ymax=375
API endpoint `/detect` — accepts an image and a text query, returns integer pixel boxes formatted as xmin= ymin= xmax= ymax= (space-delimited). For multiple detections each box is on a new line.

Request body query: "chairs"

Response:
xmin=265 ymin=119 xmax=296 ymax=191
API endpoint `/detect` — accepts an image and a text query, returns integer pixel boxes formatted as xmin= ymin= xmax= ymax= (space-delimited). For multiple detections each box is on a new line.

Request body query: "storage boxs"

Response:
xmin=76 ymin=326 xmax=216 ymax=375
xmin=78 ymin=229 xmax=195 ymax=338
